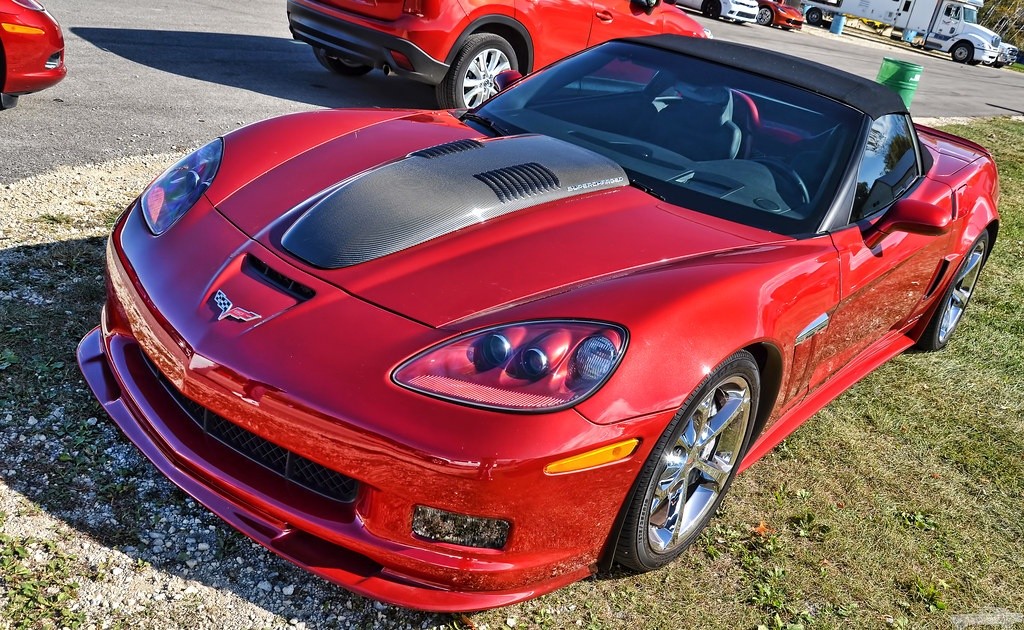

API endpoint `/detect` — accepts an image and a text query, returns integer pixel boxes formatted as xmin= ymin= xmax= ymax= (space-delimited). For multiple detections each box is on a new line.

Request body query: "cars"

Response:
xmin=285 ymin=0 xmax=720 ymax=117
xmin=663 ymin=0 xmax=762 ymax=26
xmin=0 ymin=1 xmax=69 ymax=114
xmin=756 ymin=0 xmax=806 ymax=32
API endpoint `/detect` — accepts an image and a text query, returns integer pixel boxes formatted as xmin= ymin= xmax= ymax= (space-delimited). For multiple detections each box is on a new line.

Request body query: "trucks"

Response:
xmin=980 ymin=41 xmax=1020 ymax=69
xmin=806 ymin=0 xmax=1001 ymax=66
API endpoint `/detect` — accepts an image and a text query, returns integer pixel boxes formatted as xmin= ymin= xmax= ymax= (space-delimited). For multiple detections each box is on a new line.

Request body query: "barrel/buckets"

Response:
xmin=875 ymin=57 xmax=925 ymax=113
xmin=899 ymin=29 xmax=918 ymax=43
xmin=829 ymin=12 xmax=847 ymax=35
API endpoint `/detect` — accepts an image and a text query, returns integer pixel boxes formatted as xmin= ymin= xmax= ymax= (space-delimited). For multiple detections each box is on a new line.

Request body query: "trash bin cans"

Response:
xmin=877 ymin=56 xmax=925 ymax=114
xmin=829 ymin=12 xmax=847 ymax=36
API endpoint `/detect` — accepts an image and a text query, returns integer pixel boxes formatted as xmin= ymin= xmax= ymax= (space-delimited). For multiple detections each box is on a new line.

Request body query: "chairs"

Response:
xmin=640 ymin=86 xmax=741 ymax=161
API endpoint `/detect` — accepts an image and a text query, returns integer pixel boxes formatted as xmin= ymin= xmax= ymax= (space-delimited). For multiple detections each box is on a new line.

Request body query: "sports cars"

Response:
xmin=76 ymin=34 xmax=1004 ymax=612
